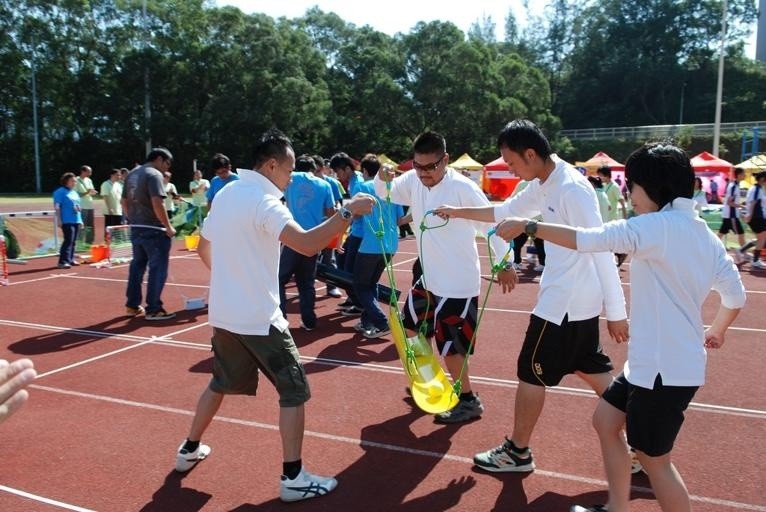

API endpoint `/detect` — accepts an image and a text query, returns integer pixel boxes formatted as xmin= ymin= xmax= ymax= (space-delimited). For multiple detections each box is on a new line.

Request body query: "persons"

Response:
xmin=586 ymin=175 xmax=611 ymax=226
xmin=716 ymin=167 xmax=754 ymax=259
xmin=493 ymin=142 xmax=746 ymax=511
xmin=121 ymin=168 xmax=129 ymax=182
xmin=597 ymin=167 xmax=629 ymax=267
xmin=121 ymin=148 xmax=176 ymax=320
xmin=693 ymin=177 xmax=708 ymax=218
xmin=373 ymin=129 xmax=518 ymax=424
xmin=205 ymin=153 xmax=240 ymax=210
xmin=272 ymin=155 xmax=339 ymax=332
xmin=353 ymin=154 xmax=413 ymax=338
xmin=176 ymin=130 xmax=375 ymax=501
xmin=74 ymin=166 xmax=98 ymax=244
xmin=323 ymin=159 xmax=346 ymax=197
xmin=735 ymin=172 xmax=766 ymax=267
xmin=710 ymin=180 xmax=719 ymax=201
xmin=311 ymin=156 xmax=342 ymax=298
xmin=190 ymin=170 xmax=210 ymax=208
xmin=100 ymin=169 xmax=124 ymax=245
xmin=328 ymin=152 xmax=365 ymax=315
xmin=432 ymin=119 xmax=644 ymax=474
xmin=54 ymin=173 xmax=84 ymax=268
xmin=615 ymin=174 xmax=622 ymax=187
xmin=164 ymin=171 xmax=178 ymax=220
xmin=725 ymin=178 xmax=731 ymax=193
xmin=1 ymin=356 xmax=36 ymax=431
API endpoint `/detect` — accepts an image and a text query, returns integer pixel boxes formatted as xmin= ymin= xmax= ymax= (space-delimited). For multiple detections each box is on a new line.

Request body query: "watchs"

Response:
xmin=339 ymin=208 xmax=356 ymax=224
xmin=524 ymin=219 xmax=539 ymax=238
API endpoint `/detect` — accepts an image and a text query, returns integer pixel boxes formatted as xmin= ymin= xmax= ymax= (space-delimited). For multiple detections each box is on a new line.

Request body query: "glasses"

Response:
xmin=625 ymin=178 xmax=636 ymax=194
xmin=412 ymin=156 xmax=446 ymax=172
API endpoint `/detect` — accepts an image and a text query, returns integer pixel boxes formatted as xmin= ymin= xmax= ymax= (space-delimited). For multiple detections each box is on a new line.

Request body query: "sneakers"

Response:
xmin=473 ymin=443 xmax=537 ymax=473
xmin=362 ymin=324 xmax=391 ymax=338
xmin=126 ymin=306 xmax=145 ymax=316
xmin=435 ymin=398 xmax=484 ymax=423
xmin=752 ymin=261 xmax=764 ymax=268
xmin=58 ymin=260 xmax=70 ymax=268
xmin=354 ymin=321 xmax=369 ymax=331
xmin=301 ymin=322 xmax=314 ymax=330
xmin=630 ymin=448 xmax=646 ymax=474
xmin=327 ymin=288 xmax=342 ymax=297
xmin=735 ymin=250 xmax=743 ymax=262
xmin=176 ymin=441 xmax=209 ymax=471
xmin=572 ymin=504 xmax=607 ymax=512
xmin=146 ymin=308 xmax=175 ymax=320
xmin=342 ymin=306 xmax=365 ymax=315
xmin=337 ymin=300 xmax=353 ymax=307
xmin=70 ymin=258 xmax=81 ymax=266
xmin=280 ymin=469 xmax=338 ymax=502
